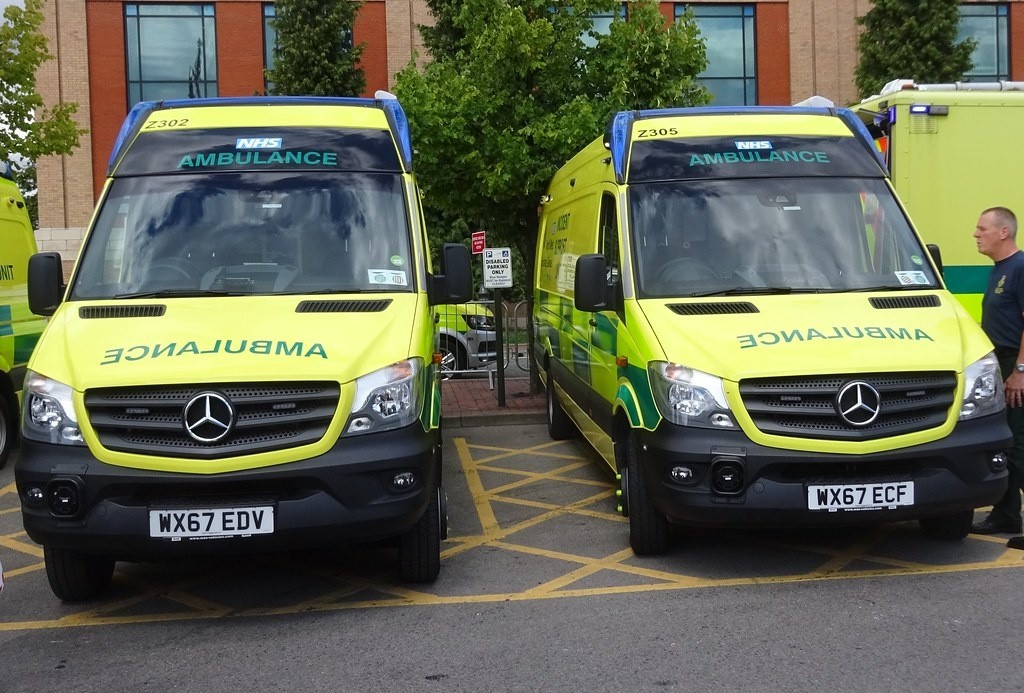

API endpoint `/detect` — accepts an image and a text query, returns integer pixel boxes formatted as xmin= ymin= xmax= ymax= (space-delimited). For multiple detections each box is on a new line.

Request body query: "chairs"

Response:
xmin=296 ymin=186 xmax=391 ymax=285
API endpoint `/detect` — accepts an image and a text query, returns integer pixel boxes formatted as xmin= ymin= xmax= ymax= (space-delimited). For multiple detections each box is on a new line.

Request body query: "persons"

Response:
xmin=970 ymin=207 xmax=1024 ymax=550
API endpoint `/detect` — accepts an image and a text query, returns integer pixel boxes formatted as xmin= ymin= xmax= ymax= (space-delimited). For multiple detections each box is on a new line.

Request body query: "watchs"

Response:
xmin=1014 ymin=363 xmax=1024 ymax=372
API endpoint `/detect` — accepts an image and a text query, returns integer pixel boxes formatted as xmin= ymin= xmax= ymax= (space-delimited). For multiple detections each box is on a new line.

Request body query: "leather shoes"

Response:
xmin=1007 ymin=537 xmax=1024 ymax=550
xmin=969 ymin=516 xmax=1022 ymax=534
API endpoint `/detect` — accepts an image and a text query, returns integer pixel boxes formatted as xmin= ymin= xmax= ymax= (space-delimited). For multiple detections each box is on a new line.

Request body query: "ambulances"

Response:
xmin=529 ymin=105 xmax=1016 ymax=558
xmin=434 ymin=299 xmax=498 ymax=380
xmin=14 ymin=90 xmax=472 ymax=604
xmin=831 ymin=76 xmax=1024 ymax=344
xmin=0 ymin=159 xmax=54 ymax=478
